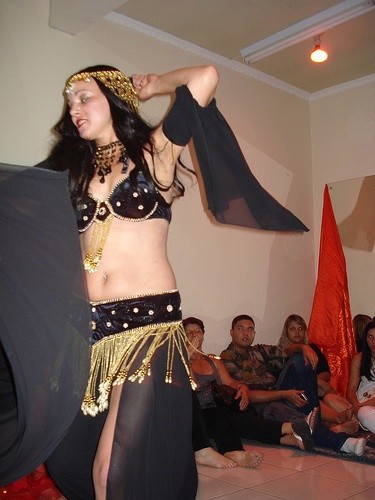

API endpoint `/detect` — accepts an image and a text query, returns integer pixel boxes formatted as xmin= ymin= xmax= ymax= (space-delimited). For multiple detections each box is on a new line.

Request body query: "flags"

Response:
xmin=306 ymin=181 xmax=356 ymax=402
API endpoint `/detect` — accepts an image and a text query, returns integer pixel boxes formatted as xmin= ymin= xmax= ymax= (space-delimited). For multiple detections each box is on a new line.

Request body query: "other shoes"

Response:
xmin=339 ymin=437 xmax=366 ymax=457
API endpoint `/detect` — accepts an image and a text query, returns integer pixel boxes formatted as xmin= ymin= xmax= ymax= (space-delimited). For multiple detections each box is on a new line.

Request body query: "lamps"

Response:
xmin=310 ymin=34 xmax=328 ymax=63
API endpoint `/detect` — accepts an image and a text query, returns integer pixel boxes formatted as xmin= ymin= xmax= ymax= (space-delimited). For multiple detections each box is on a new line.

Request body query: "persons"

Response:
xmin=31 ymin=63 xmax=219 ymax=500
xmin=182 ymin=312 xmax=375 ymax=468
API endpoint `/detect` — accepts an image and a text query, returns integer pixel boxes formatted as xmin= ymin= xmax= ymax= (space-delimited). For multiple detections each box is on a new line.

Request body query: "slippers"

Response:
xmin=292 ymin=407 xmax=319 ymax=452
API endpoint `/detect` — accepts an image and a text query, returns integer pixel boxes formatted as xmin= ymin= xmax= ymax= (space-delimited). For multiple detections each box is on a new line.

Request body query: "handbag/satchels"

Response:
xmin=196 ymin=380 xmax=224 ymax=410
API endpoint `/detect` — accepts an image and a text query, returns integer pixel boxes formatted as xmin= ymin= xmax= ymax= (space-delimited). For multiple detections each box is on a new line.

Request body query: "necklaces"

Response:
xmin=92 ymin=141 xmax=129 ymax=184
